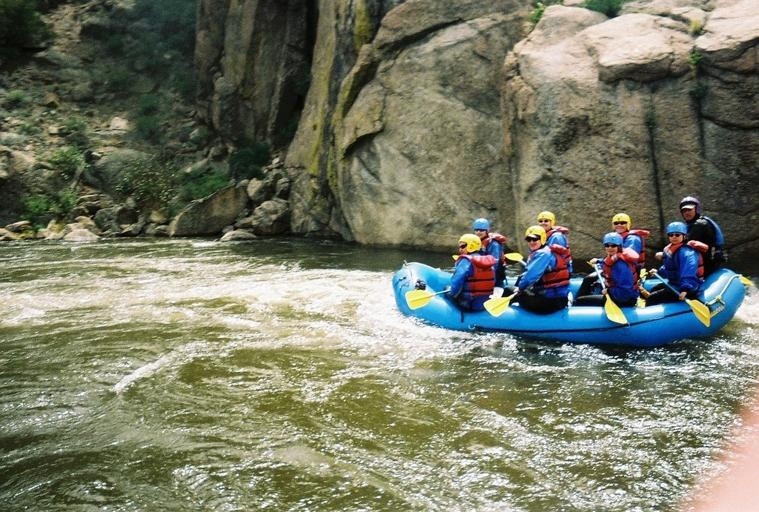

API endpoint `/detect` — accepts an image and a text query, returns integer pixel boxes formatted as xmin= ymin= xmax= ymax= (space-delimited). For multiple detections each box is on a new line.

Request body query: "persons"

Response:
xmin=536 ymin=211 xmax=574 ymax=284
xmin=611 ymin=213 xmax=645 ymax=297
xmin=444 ymin=233 xmax=495 ymax=313
xmin=472 ymin=218 xmax=508 ymax=296
xmin=645 ymin=220 xmax=698 ymax=305
xmin=654 ymin=197 xmax=723 ymax=278
xmin=574 ymin=232 xmax=640 ymax=307
xmin=515 ymin=226 xmax=568 ymax=314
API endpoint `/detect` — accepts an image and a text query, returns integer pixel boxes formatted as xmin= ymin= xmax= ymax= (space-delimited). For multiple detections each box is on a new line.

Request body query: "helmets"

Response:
xmin=679 ymin=196 xmax=700 ymax=210
xmin=537 ymin=211 xmax=555 ymax=221
xmin=472 ymin=218 xmax=489 ymax=230
xmin=602 ymin=232 xmax=623 ymax=247
xmin=612 ymin=213 xmax=631 ymax=222
xmin=458 ymin=234 xmax=481 ymax=244
xmin=666 ymin=222 xmax=688 ymax=235
xmin=524 ymin=225 xmax=546 ymax=239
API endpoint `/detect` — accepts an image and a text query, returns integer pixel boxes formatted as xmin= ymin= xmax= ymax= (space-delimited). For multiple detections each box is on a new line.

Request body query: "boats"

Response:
xmin=391 ymin=259 xmax=746 ymax=344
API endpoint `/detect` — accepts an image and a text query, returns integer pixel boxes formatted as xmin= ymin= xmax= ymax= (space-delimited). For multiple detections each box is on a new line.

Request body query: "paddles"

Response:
xmin=652 ymin=272 xmax=710 ymax=328
xmin=586 ymin=261 xmax=628 ymax=324
xmin=504 ymin=252 xmax=529 ymax=270
xmin=482 ymin=293 xmax=518 ymax=317
xmin=405 ymin=290 xmax=449 ymax=310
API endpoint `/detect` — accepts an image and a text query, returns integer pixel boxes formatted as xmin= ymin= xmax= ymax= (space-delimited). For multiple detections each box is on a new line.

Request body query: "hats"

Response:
xmin=681 ymin=204 xmax=696 ymax=210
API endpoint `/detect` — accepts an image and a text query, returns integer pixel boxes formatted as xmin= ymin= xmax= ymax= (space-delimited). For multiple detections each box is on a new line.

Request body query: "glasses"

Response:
xmin=459 ymin=244 xmax=466 ymax=248
xmin=669 ymin=233 xmax=682 ymax=237
xmin=614 ymin=221 xmax=627 ymax=225
xmin=526 ymin=238 xmax=539 ymax=242
xmin=604 ymin=244 xmax=616 ymax=248
